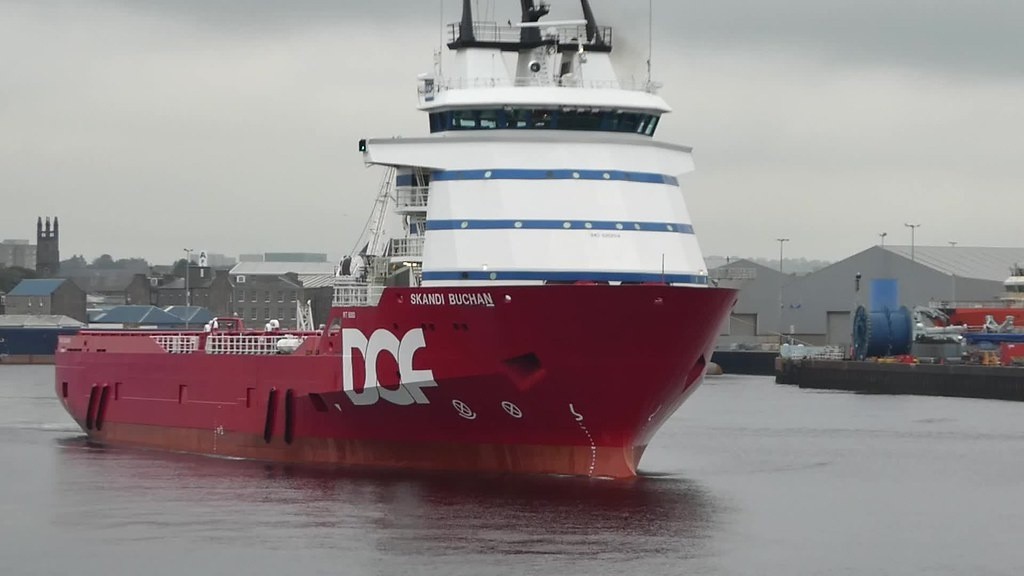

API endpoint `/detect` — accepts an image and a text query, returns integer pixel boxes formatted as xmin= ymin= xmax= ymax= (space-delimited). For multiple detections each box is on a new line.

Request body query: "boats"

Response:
xmin=54 ymin=0 xmax=743 ymax=494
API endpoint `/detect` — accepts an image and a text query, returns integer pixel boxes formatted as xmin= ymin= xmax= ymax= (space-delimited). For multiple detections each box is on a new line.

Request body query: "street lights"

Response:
xmin=903 ymin=223 xmax=924 ymax=261
xmin=183 ymin=248 xmax=193 ymax=333
xmin=777 ymin=237 xmax=789 ymax=349
xmin=878 ymin=232 xmax=890 ymax=247
